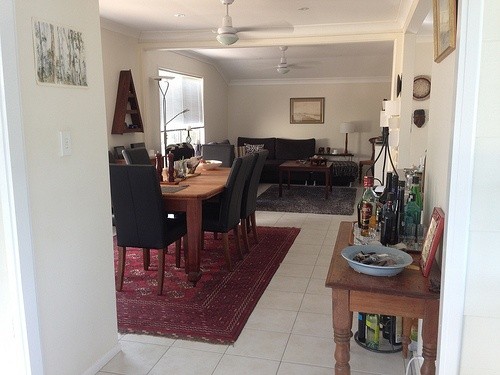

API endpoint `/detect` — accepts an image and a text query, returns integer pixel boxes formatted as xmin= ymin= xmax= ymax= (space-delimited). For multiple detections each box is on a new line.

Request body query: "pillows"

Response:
xmin=246 ymin=144 xmax=265 ymax=154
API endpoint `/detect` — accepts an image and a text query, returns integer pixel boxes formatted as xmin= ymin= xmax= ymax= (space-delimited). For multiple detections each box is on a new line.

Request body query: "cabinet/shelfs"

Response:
xmin=151 ymin=156 xmax=164 ymax=175
xmin=373 ymin=145 xmax=386 ymax=190
xmin=111 ymin=70 xmax=144 ymax=134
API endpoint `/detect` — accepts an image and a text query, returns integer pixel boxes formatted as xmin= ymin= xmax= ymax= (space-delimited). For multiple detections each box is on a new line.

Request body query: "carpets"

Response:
xmin=112 ymin=225 xmax=302 ymax=343
xmin=256 ymin=183 xmax=357 ymax=216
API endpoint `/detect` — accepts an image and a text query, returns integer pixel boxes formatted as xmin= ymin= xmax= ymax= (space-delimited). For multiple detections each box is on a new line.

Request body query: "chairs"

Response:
xmin=109 ymin=143 xmax=270 ymax=295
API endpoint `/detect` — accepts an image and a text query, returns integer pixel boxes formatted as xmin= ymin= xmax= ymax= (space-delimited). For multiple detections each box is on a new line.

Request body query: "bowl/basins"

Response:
xmin=340 ymin=244 xmax=413 ymax=276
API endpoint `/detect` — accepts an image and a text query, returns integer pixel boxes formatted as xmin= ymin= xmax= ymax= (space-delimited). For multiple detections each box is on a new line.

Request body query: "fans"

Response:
xmin=256 ymin=45 xmax=318 ymax=73
xmin=150 ymin=0 xmax=295 ymax=46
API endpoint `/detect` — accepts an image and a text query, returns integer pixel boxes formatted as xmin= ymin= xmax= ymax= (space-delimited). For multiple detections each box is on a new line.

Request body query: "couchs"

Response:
xmin=238 ymin=137 xmax=317 ymax=185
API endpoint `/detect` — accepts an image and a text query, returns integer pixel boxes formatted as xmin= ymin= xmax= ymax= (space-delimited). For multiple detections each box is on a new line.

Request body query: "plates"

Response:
xmin=196 ymin=159 xmax=223 ymax=170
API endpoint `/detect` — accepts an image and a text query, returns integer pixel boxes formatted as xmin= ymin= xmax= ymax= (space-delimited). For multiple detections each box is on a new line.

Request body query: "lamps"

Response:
xmin=365 ymin=111 xmax=399 ymax=185
xmin=151 ymin=74 xmax=190 ymax=167
xmin=186 ymin=125 xmax=192 ymax=143
xmin=337 ymin=122 xmax=356 ymax=156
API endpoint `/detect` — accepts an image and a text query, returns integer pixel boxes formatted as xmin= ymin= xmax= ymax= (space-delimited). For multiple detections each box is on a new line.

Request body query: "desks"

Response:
xmin=279 ymin=161 xmax=333 ymax=200
xmin=325 ymin=220 xmax=441 ymax=375
xmin=161 ymin=167 xmax=230 ymax=284
xmin=312 ymin=153 xmax=355 ymax=187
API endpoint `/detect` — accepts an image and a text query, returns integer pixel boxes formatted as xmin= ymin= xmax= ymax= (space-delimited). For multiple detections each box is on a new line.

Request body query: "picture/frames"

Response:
xmin=290 ymin=98 xmax=324 ymax=124
xmin=420 ymin=207 xmax=445 ymax=277
xmin=433 ymin=0 xmax=457 ymax=63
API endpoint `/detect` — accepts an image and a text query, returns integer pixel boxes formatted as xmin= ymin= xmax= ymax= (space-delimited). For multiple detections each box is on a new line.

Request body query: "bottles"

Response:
xmin=154 ymin=153 xmax=163 ymax=181
xmin=166 ymin=153 xmax=174 ymax=182
xmin=356 ymin=171 xmax=423 ymax=246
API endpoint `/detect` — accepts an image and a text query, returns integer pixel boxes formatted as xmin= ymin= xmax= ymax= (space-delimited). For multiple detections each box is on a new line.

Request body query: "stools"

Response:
xmin=358 ymin=160 xmax=374 ymax=184
